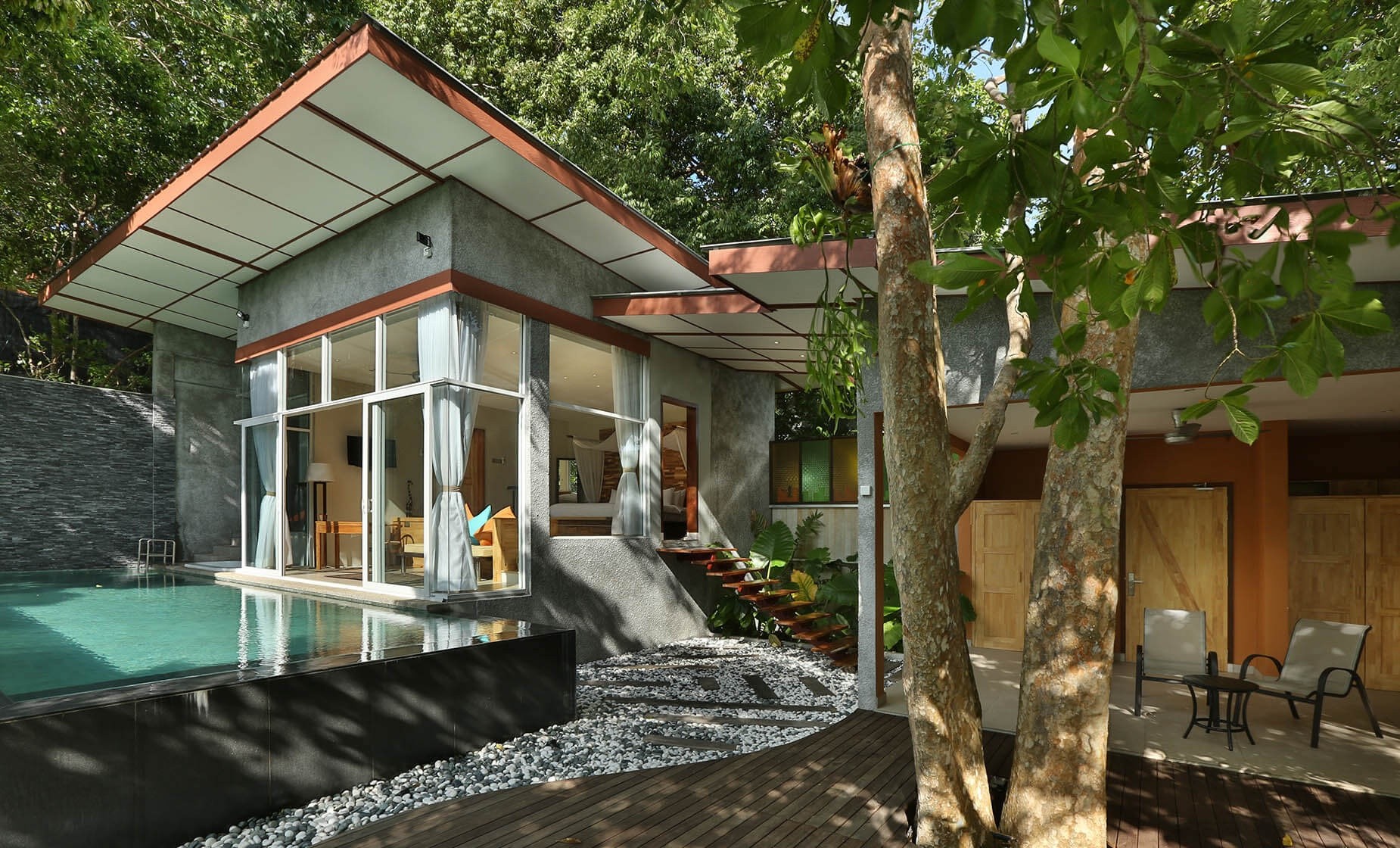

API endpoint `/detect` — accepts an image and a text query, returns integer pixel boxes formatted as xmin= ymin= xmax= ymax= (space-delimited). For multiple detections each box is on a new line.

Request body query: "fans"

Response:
xmin=370 ymin=369 xmax=420 ymax=383
xmin=1123 ymin=407 xmax=1270 ymax=443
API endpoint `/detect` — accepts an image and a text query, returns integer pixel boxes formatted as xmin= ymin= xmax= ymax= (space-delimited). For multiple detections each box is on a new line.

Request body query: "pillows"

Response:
xmin=609 ymin=489 xmax=618 ymax=503
xmin=464 ymin=503 xmax=517 ymax=545
xmin=661 ymin=488 xmax=686 ymax=506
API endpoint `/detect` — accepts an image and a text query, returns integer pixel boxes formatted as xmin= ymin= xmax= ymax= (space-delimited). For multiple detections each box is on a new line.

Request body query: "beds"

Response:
xmin=550 ymin=503 xmax=686 ymax=536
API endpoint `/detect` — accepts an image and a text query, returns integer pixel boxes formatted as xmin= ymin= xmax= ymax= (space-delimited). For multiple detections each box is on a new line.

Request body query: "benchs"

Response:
xmin=398 ymin=534 xmax=493 ymax=581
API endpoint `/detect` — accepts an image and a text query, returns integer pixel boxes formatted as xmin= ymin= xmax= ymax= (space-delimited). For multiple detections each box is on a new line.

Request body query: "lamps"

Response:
xmin=236 ymin=310 xmax=251 ymax=329
xmin=304 ymin=463 xmax=335 ymax=568
xmin=417 ymin=231 xmax=433 ymax=258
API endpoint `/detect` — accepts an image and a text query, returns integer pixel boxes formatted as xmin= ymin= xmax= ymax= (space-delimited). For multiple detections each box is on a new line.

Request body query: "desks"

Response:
xmin=1183 ymin=675 xmax=1259 ymax=750
xmin=393 ymin=518 xmax=424 ymax=568
xmin=314 ymin=520 xmax=370 ymax=570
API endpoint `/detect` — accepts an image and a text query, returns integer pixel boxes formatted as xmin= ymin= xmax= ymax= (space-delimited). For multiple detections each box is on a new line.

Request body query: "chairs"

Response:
xmin=1134 ymin=607 xmax=1220 ymax=728
xmin=1232 ymin=618 xmax=1384 ymax=746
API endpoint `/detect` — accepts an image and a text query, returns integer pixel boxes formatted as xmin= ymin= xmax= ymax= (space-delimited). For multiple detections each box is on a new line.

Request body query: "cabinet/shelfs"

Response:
xmin=433 ymin=428 xmax=485 ymax=516
